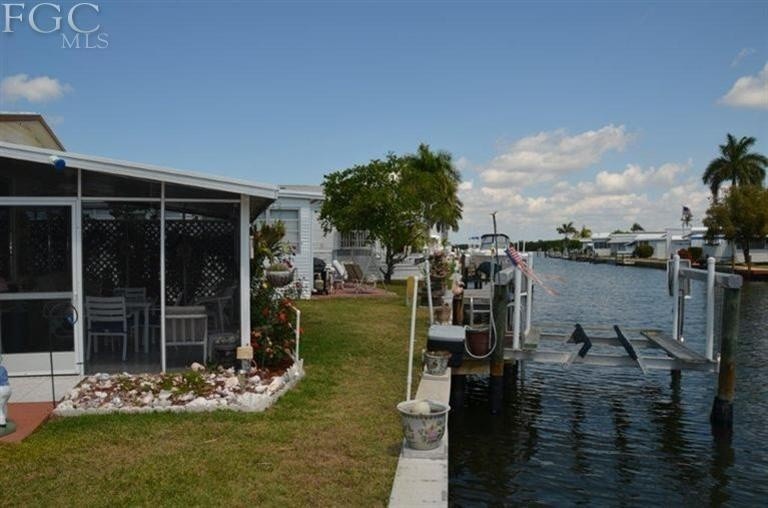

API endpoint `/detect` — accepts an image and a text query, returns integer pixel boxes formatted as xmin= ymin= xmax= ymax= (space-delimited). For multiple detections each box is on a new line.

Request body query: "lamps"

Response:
xmin=48 ymin=154 xmax=66 ymax=172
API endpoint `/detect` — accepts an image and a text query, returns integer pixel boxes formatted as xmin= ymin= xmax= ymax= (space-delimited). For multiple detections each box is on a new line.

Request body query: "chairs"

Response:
xmin=331 ymin=259 xmax=377 ymax=293
xmin=32 ymin=274 xmax=237 ymax=367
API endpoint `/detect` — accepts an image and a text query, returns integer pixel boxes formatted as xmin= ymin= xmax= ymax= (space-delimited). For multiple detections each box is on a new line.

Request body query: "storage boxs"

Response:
xmin=426 ymin=324 xmax=465 ymax=368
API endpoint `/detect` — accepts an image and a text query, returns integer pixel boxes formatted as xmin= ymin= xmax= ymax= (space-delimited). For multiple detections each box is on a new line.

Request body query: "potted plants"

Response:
xmin=259 ymin=239 xmax=299 ymax=288
xmin=424 ymin=349 xmax=452 ymax=375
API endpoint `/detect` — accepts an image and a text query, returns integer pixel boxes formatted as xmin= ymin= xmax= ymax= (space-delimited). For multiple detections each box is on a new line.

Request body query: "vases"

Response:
xmin=395 ymin=399 xmax=450 ymax=451
xmin=467 ymin=328 xmax=489 ymax=355
